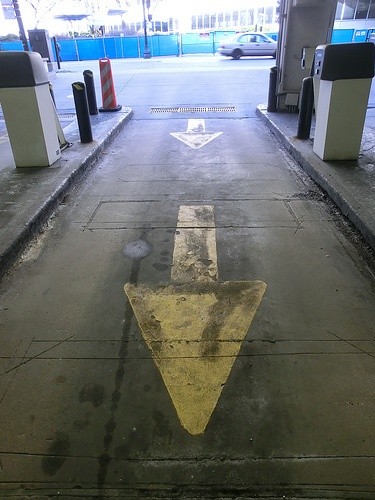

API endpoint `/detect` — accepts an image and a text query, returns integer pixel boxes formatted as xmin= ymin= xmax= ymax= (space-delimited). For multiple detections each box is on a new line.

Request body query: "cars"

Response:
xmin=217 ymin=32 xmax=278 ymax=60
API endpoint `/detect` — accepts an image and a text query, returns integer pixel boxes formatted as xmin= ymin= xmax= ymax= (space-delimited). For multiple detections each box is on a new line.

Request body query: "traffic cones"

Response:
xmin=98 ymin=57 xmax=122 ymax=112
